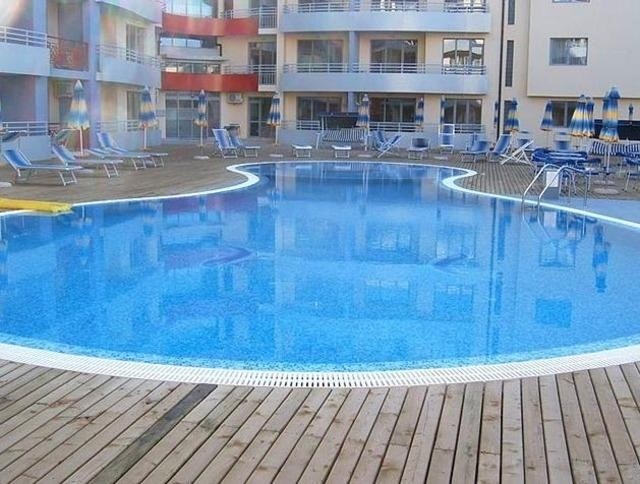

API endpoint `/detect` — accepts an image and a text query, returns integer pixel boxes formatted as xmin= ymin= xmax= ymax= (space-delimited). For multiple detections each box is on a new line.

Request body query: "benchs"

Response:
xmin=291 ymin=126 xmax=370 ymax=158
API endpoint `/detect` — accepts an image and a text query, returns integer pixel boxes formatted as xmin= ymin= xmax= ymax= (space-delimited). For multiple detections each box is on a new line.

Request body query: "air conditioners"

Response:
xmin=226 ymin=92 xmax=243 ymax=103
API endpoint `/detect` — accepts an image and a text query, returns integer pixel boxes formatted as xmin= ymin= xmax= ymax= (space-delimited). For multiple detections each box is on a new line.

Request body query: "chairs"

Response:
xmin=211 ymin=127 xmax=260 ymax=158
xmin=1 ymin=127 xmax=168 ymax=185
xmin=372 ymin=129 xmax=535 ymax=165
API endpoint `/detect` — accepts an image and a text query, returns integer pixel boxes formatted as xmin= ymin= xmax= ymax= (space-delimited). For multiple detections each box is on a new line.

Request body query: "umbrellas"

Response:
xmin=568 ymin=86 xmax=621 ymax=175
xmin=540 ymin=99 xmax=553 ymax=147
xmin=593 ymin=221 xmax=609 ymax=293
xmin=70 ymin=207 xmax=92 ymax=266
xmin=504 ymin=97 xmax=519 ymax=146
xmin=138 ymin=83 xmax=158 ymax=148
xmin=67 ymin=79 xmax=91 ymax=156
xmin=266 ymin=87 xmax=283 ymax=154
xmin=194 ymin=88 xmax=209 ymax=156
xmin=139 ymin=200 xmax=162 ymax=238
xmin=199 ymin=195 xmax=207 ymax=221
xmin=356 ymin=94 xmax=371 ymax=152
xmin=0 ymin=223 xmax=10 ymax=296
xmin=416 ymin=96 xmax=425 ymax=137
